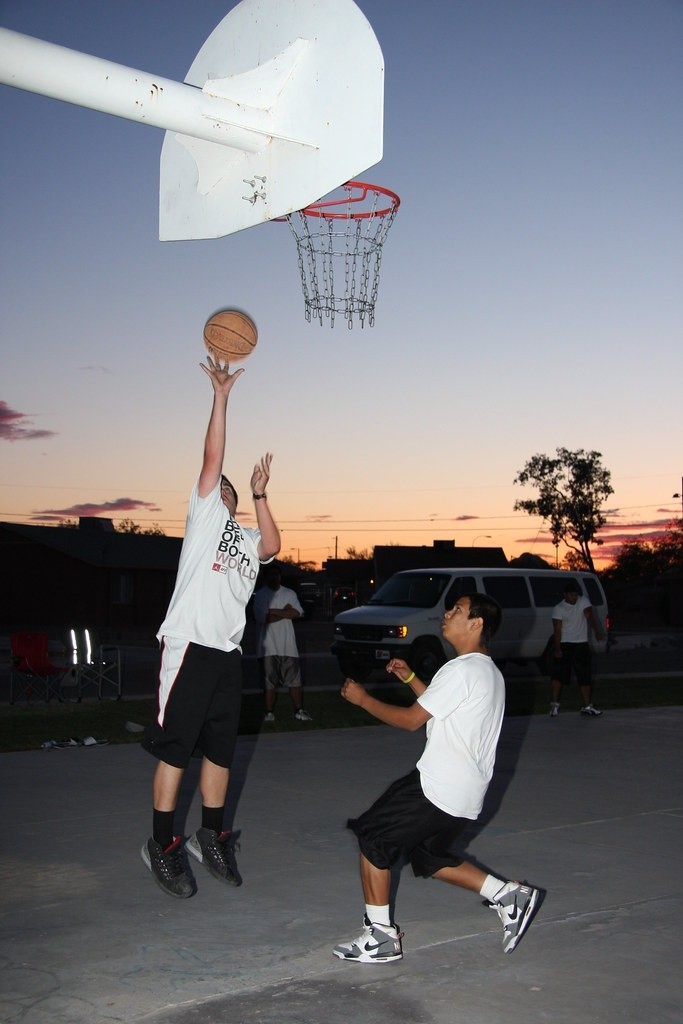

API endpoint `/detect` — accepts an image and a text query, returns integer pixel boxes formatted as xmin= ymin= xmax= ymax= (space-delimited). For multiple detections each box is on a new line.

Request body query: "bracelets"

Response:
xmin=403 ymin=672 xmax=415 ymax=683
xmin=253 ymin=493 xmax=266 ymax=500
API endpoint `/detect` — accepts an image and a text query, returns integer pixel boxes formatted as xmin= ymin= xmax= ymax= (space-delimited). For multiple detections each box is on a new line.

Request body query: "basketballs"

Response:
xmin=203 ymin=310 xmax=260 ymax=364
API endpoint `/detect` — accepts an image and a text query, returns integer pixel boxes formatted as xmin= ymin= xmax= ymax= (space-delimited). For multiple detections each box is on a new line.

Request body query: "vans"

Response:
xmin=328 ymin=568 xmax=610 ymax=683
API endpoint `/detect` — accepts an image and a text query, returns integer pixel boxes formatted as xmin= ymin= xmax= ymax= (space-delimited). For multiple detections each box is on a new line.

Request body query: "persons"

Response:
xmin=141 ymin=355 xmax=282 ymax=898
xmin=332 ymin=593 xmax=539 ymax=962
xmin=253 ymin=564 xmax=314 ymax=721
xmin=549 ymin=584 xmax=603 ymax=716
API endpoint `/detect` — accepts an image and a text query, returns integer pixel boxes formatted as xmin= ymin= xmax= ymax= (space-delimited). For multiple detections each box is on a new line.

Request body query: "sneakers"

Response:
xmin=293 ymin=709 xmax=313 ymax=722
xmin=550 ymin=702 xmax=559 ymax=716
xmin=482 ymin=880 xmax=540 ymax=954
xmin=141 ymin=836 xmax=195 ymax=899
xmin=581 ymin=704 xmax=603 ymax=717
xmin=333 ymin=914 xmax=403 ymax=963
xmin=264 ymin=711 xmax=275 ymax=722
xmin=185 ymin=828 xmax=243 ymax=887
